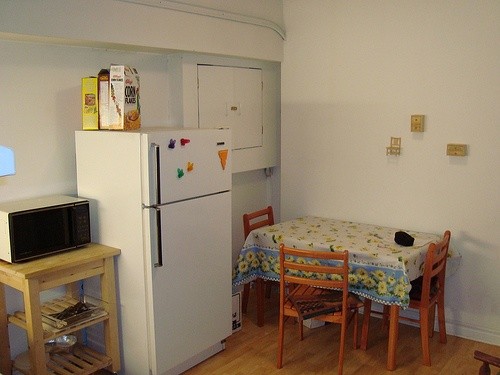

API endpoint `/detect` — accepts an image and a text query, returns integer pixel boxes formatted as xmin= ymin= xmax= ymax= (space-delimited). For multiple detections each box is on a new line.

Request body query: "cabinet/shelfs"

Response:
xmin=0 ymin=242 xmax=121 ymax=375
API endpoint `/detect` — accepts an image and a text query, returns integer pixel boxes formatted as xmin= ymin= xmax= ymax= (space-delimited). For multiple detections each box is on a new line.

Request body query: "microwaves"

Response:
xmin=0 ymin=195 xmax=91 ymax=264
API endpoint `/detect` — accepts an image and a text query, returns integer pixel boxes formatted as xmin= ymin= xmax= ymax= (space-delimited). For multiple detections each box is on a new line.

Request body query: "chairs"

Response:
xmin=242 ymin=205 xmax=295 ymax=313
xmin=276 ymin=244 xmax=359 ymax=375
xmin=360 ymin=230 xmax=452 ymax=367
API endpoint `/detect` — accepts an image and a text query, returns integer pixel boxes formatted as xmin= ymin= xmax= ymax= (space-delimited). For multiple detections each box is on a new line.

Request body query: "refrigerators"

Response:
xmin=75 ymin=128 xmax=233 ymax=375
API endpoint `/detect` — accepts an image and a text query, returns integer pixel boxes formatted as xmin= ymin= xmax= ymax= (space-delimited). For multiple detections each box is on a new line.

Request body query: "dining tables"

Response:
xmin=232 ymin=216 xmax=442 ymax=370
